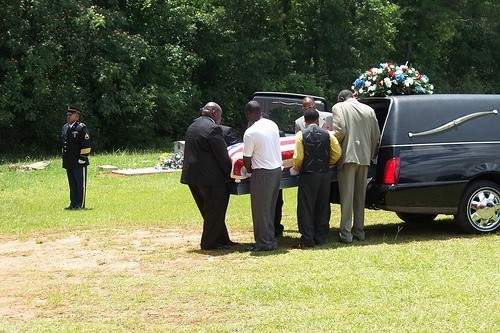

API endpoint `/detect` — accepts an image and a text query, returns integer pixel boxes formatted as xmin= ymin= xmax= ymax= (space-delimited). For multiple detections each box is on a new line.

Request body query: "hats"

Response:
xmin=66 ymin=106 xmax=82 ymax=115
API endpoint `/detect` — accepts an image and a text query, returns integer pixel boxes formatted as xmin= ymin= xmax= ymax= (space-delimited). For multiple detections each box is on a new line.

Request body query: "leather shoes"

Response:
xmin=246 ymin=246 xmax=275 ymax=252
xmin=215 ymin=245 xmax=231 ymax=249
xmin=291 ymin=241 xmax=312 ymax=248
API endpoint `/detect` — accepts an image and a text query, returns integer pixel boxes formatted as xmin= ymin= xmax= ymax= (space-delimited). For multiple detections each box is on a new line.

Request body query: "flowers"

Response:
xmin=158 ymin=149 xmax=183 ymax=170
xmin=352 ymin=61 xmax=434 ymax=99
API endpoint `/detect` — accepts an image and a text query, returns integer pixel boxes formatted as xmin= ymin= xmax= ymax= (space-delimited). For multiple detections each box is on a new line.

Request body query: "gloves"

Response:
xmin=78 ymin=159 xmax=86 ymax=164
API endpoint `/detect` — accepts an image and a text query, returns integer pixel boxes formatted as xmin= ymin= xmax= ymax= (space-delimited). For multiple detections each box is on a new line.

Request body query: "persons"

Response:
xmin=289 ymin=107 xmax=342 ymax=247
xmin=330 ymin=90 xmax=380 ymax=243
xmin=61 ymin=104 xmax=91 ymax=210
xmin=295 ymin=96 xmax=334 ymax=133
xmin=181 ymin=101 xmax=238 ymax=250
xmin=258 ymin=111 xmax=286 ymax=237
xmin=241 ymin=100 xmax=284 ymax=251
xmin=196 ymin=107 xmax=239 ymax=148
